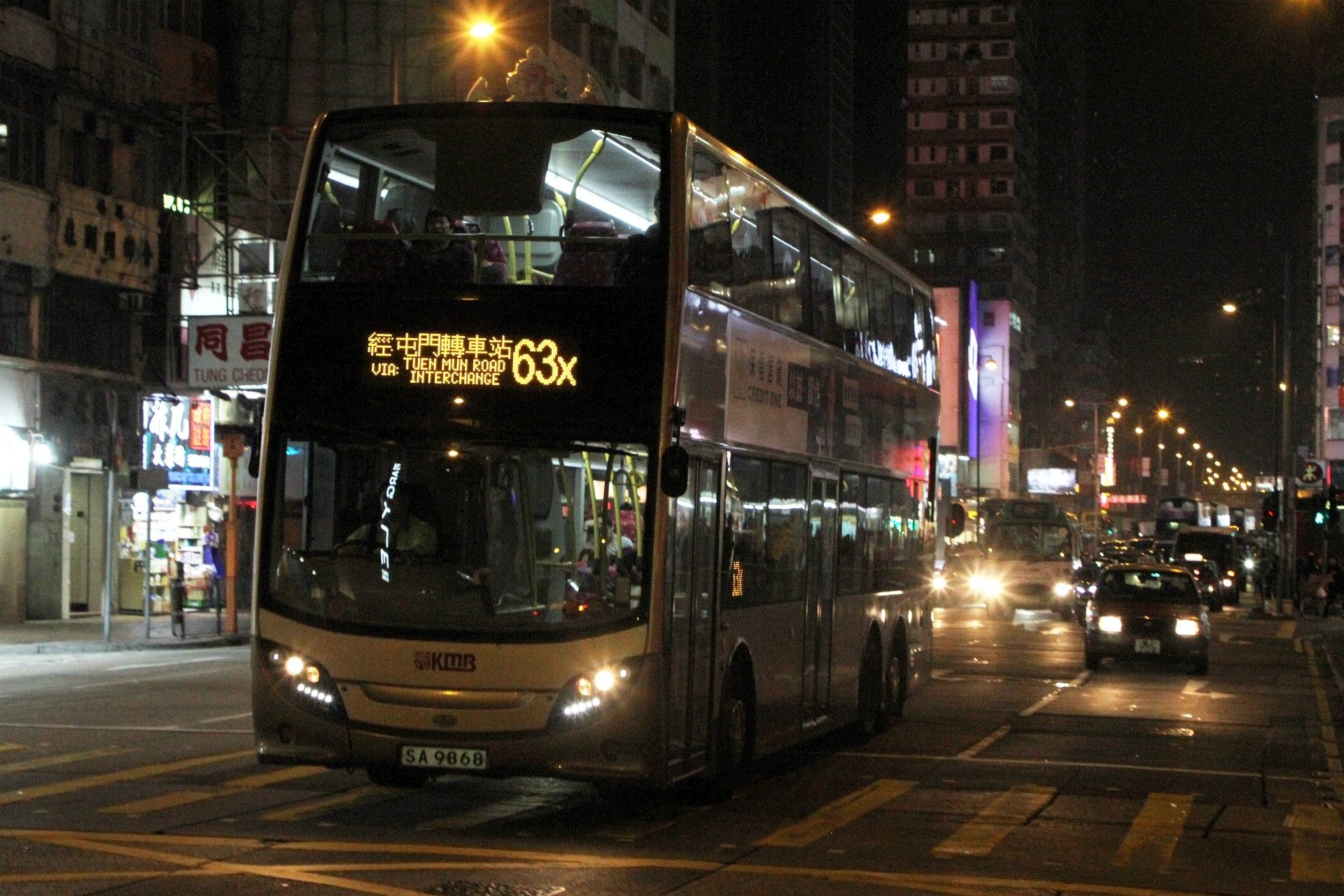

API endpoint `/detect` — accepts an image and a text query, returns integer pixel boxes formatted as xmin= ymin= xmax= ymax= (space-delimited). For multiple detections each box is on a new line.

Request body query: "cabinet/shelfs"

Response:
xmin=120 ymin=506 xmax=211 ymax=614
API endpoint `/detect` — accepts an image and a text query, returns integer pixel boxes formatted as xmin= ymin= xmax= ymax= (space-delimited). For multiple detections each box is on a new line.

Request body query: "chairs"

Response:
xmin=339 ymin=218 xmax=506 ymax=284
xmin=550 ymin=220 xmax=838 ymax=345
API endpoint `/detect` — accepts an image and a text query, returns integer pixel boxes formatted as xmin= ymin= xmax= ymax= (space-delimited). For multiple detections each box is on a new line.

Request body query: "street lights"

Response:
xmin=392 ymin=21 xmax=498 ymax=107
xmin=1220 ymin=299 xmax=1280 ymax=490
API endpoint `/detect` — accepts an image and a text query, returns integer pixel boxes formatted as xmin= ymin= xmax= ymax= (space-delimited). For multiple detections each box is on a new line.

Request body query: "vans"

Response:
xmin=977 ymin=500 xmax=1081 ymax=623
xmin=953 ymin=494 xmax=1277 ymax=680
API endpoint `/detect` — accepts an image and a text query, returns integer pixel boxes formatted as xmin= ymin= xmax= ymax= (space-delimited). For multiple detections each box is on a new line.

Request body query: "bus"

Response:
xmin=246 ymin=99 xmax=944 ymax=809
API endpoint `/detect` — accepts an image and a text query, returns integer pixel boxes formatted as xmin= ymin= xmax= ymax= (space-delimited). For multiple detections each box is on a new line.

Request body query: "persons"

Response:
xmin=1252 ymin=548 xmax=1280 ymax=600
xmin=203 ymin=534 xmax=218 ymax=607
xmin=344 ymin=206 xmax=509 ymax=298
xmin=1301 ymin=551 xmax=1342 ymax=620
xmin=338 ymin=476 xmax=437 ymax=566
xmin=645 ymin=189 xmax=661 ymax=238
xmin=582 ymin=502 xmax=644 ymax=590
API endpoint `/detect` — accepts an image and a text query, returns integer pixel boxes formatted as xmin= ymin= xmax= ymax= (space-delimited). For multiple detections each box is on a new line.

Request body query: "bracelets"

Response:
xmin=583 ymin=528 xmax=587 ymax=532
